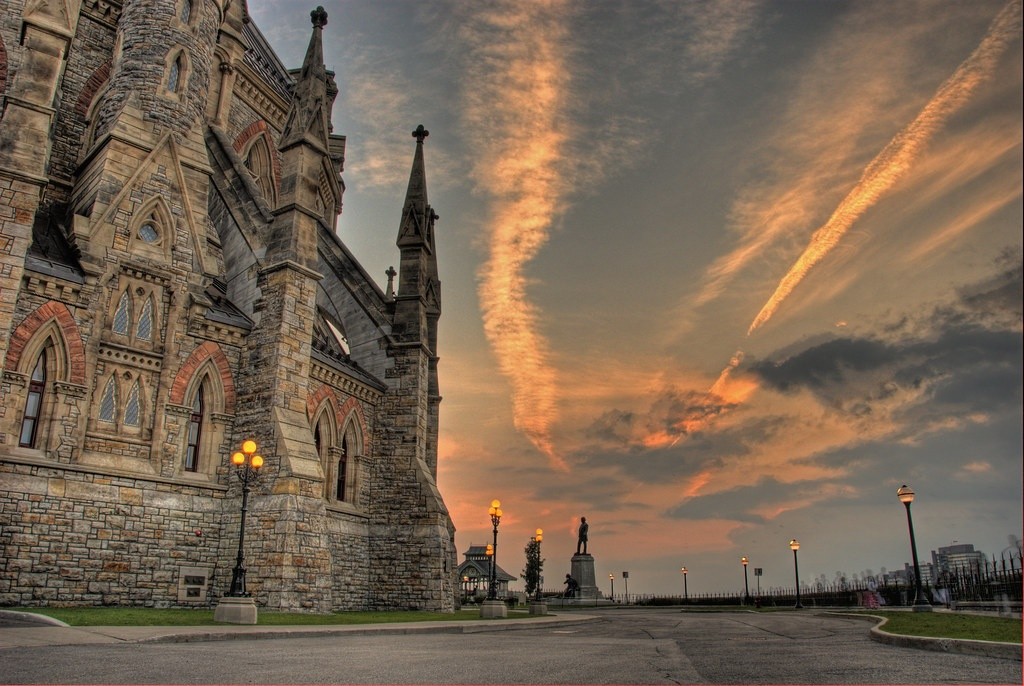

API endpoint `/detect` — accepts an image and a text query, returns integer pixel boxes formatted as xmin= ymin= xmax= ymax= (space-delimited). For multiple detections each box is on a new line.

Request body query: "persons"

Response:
xmin=564 ymin=573 xmax=578 ymax=598
xmin=575 ymin=517 xmax=588 ymax=554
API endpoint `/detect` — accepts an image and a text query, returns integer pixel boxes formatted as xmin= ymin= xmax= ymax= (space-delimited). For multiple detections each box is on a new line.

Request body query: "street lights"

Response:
xmin=681 ymin=567 xmax=689 ymax=599
xmin=897 ymin=484 xmax=934 ymax=612
xmin=485 ymin=544 xmax=494 ymax=600
xmin=609 ymin=573 xmax=614 ymax=598
xmin=214 ymin=440 xmax=265 ymax=625
xmin=742 ymin=556 xmax=749 ymax=596
xmin=463 ymin=576 xmax=469 ymax=606
xmin=789 ymin=538 xmax=805 ymax=611
xmin=480 ymin=499 xmax=507 ymax=619
xmin=529 ymin=528 xmax=547 ymax=615
